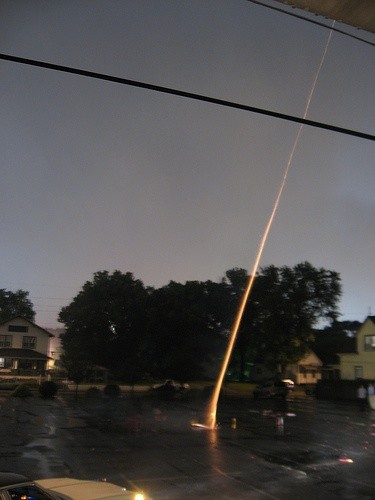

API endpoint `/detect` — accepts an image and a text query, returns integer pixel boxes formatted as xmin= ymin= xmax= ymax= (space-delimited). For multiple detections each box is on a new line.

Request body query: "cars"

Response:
xmin=251 ymin=378 xmax=294 ymax=399
